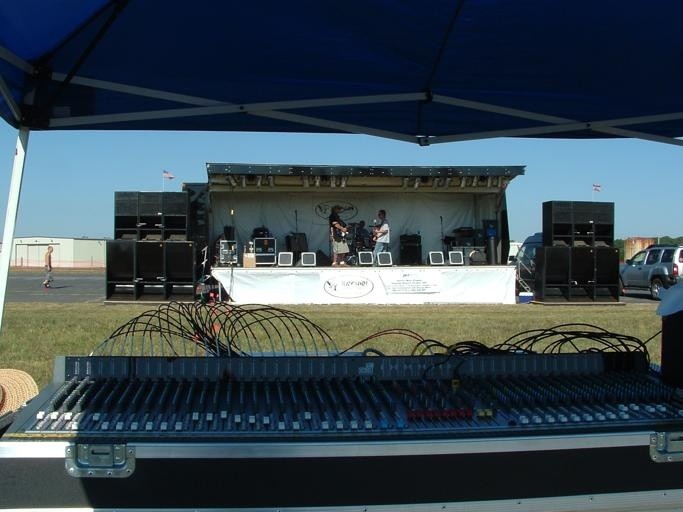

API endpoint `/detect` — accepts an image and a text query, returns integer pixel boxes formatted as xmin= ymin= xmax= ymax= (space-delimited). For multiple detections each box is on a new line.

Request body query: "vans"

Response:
xmin=507 ymin=232 xmax=543 ymax=293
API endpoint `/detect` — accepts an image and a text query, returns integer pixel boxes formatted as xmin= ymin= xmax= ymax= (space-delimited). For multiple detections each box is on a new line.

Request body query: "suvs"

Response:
xmin=619 ymin=244 xmax=683 ymax=300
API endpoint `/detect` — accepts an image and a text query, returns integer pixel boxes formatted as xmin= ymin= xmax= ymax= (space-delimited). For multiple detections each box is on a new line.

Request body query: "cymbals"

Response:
xmin=348 ymin=223 xmax=360 ymax=226
xmin=369 ymin=225 xmax=381 ymax=227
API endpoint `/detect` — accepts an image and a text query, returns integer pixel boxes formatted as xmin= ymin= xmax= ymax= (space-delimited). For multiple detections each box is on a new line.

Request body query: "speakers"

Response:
xmin=429 ymin=251 xmax=444 ymax=266
xmin=534 ymin=201 xmax=619 ymax=303
xmin=301 ymin=252 xmax=317 ymax=267
xmin=358 ymin=251 xmax=374 ymax=265
xmin=105 ymin=191 xmax=199 ymax=305
xmin=285 ymin=233 xmax=308 ymax=252
xmin=278 ymin=252 xmax=294 ymax=267
xmin=449 ymin=251 xmax=464 ymax=265
xmin=400 ymin=243 xmax=422 ymax=265
xmin=377 ymin=252 xmax=393 ymax=267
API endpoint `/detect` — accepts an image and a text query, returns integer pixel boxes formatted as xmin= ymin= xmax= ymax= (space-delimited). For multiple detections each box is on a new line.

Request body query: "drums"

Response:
xmin=356 ymin=235 xmax=376 ymax=251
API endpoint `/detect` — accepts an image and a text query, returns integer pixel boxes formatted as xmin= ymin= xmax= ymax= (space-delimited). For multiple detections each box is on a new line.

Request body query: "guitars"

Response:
xmin=335 ymin=224 xmax=352 ymax=238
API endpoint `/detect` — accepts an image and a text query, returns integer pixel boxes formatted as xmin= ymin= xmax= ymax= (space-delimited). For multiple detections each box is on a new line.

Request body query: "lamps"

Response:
xmin=299 ymin=174 xmax=350 ymax=187
xmin=225 ymin=173 xmax=275 ymax=188
xmin=400 ymin=176 xmax=503 ymax=189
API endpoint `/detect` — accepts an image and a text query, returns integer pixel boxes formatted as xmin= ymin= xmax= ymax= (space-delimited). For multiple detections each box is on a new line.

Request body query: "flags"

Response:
xmin=592 ymin=184 xmax=600 ymax=192
xmin=161 ymin=170 xmax=176 ymax=180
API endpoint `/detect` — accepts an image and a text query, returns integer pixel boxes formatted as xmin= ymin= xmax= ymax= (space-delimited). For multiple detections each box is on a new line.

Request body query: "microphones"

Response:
xmin=373 ymin=219 xmax=377 ymax=225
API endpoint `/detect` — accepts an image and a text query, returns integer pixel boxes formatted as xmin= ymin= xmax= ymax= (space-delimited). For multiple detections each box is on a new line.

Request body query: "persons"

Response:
xmin=328 ymin=205 xmax=352 ymax=267
xmin=371 ymin=208 xmax=389 ymax=267
xmin=355 ymin=220 xmax=369 ymax=238
xmin=41 ymin=246 xmax=54 ymax=289
